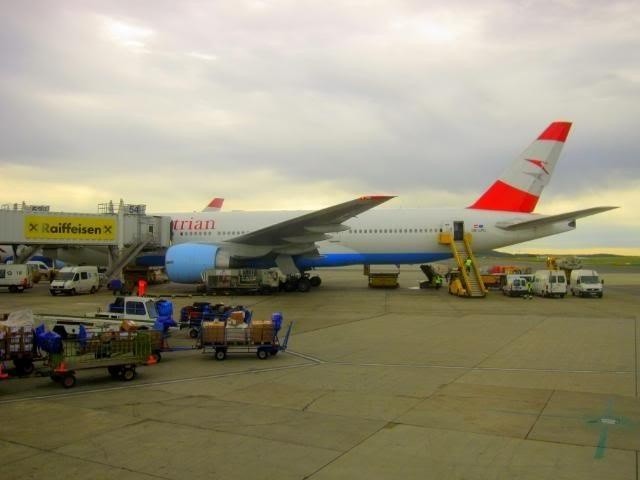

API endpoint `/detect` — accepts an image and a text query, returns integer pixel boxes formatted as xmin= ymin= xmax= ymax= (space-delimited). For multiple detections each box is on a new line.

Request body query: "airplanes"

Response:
xmin=0 ymin=120 xmax=622 ymax=292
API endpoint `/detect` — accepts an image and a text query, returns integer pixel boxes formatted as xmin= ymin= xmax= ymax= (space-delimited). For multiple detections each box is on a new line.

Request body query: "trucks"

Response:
xmin=480 ymin=256 xmax=572 ymax=289
xmin=4 ymin=294 xmax=172 ymax=339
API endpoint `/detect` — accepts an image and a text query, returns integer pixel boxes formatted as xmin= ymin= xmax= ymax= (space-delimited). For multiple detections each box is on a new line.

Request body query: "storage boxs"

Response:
xmin=122 ymin=319 xmax=139 ymax=332
xmin=202 ymin=310 xmax=275 ymax=343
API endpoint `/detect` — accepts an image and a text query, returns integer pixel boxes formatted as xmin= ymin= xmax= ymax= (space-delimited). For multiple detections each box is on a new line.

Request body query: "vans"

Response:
xmin=6 ymin=259 xmax=48 ymax=280
xmin=570 ymin=269 xmax=605 ymax=299
xmin=0 ymin=263 xmax=36 ymax=294
xmin=530 ymin=269 xmax=569 ymax=298
xmin=502 ymin=277 xmax=528 ymax=297
xmin=48 ymin=265 xmax=101 ymax=296
